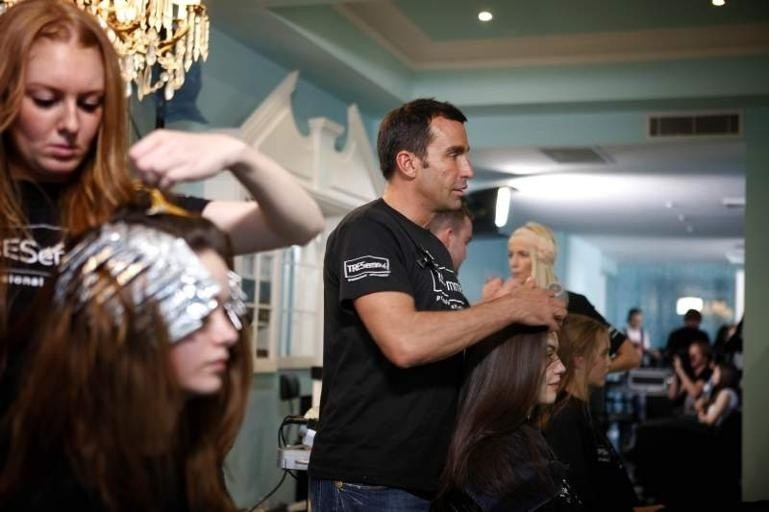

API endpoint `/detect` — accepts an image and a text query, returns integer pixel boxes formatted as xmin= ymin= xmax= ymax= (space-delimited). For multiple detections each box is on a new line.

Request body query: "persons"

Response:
xmin=0 ymin=184 xmax=253 ymax=511
xmin=440 ymin=321 xmax=579 ymax=512
xmin=0 ymin=0 xmax=323 ymax=427
xmin=307 ymin=99 xmax=567 ymax=512
xmin=427 ymin=198 xmax=473 ymax=273
